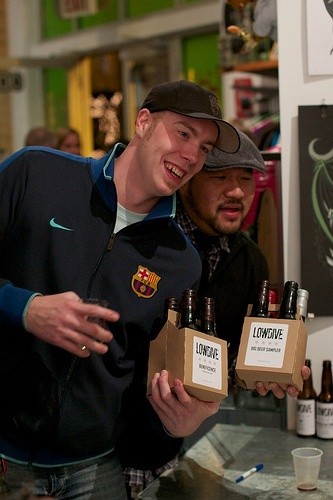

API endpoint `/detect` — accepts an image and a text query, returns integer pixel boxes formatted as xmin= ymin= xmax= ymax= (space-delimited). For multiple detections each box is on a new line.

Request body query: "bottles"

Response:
xmin=316 ymin=360 xmax=333 ymax=440
xmin=296 ymin=359 xmax=317 ymax=438
xmin=162 ymin=297 xmax=179 ymax=327
xmin=202 ymin=297 xmax=218 ymax=337
xmin=247 ymin=274 xmax=310 ymax=325
xmin=178 ymin=288 xmax=202 ymax=332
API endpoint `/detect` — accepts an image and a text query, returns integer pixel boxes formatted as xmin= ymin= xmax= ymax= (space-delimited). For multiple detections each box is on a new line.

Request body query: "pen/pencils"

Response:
xmin=236 ymin=464 xmax=263 ymax=483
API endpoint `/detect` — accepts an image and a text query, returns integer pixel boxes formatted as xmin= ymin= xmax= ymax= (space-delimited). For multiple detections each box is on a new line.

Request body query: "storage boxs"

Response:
xmin=235 ymin=304 xmax=308 ymax=391
xmin=146 ymin=310 xmax=228 ymax=403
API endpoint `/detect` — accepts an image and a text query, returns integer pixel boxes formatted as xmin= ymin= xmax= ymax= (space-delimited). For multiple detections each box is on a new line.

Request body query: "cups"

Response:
xmin=78 ymin=298 xmax=110 ymax=345
xmin=291 ymin=447 xmax=323 ymax=492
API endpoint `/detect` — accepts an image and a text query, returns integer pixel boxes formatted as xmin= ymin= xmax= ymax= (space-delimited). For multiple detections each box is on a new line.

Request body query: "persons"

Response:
xmin=0 ymin=79 xmax=310 ymax=500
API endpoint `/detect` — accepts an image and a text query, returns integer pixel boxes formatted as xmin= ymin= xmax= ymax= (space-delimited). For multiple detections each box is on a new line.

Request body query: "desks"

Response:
xmin=135 ymin=405 xmax=333 ymax=500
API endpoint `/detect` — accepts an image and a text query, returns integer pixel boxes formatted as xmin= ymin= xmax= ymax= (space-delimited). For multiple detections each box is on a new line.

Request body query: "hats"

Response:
xmin=140 ymin=79 xmax=241 ymax=154
xmin=203 ymin=127 xmax=266 ymax=174
